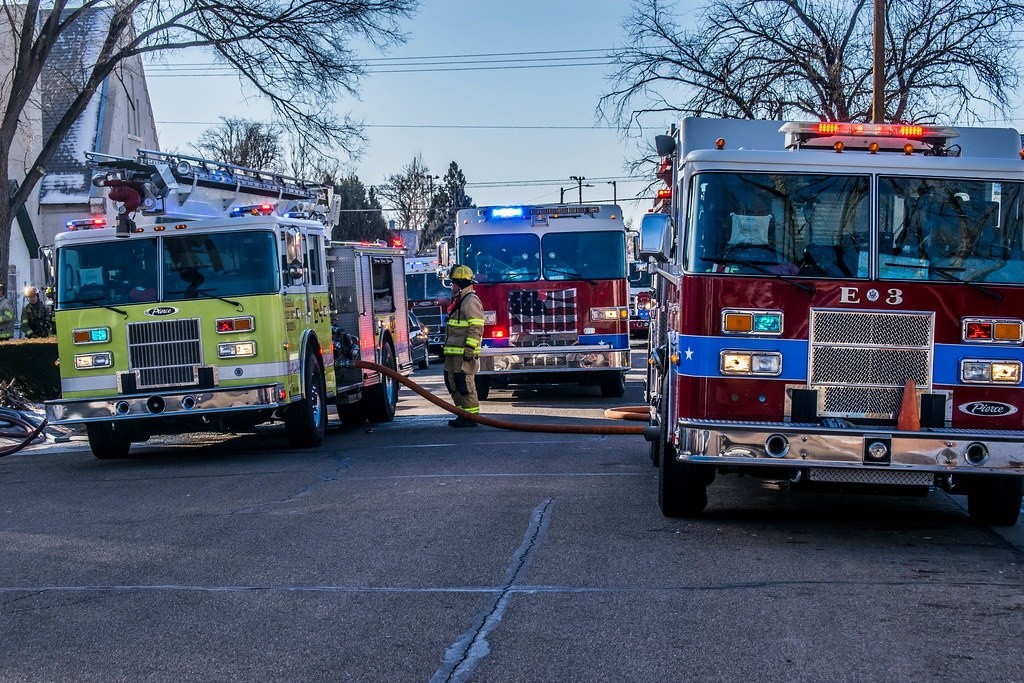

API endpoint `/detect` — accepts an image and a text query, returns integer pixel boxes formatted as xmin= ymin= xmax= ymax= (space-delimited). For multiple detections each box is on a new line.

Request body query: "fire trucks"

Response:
xmin=36 ymin=148 xmax=413 ymax=460
xmin=402 ymin=246 xmax=456 ymax=359
xmin=639 ymin=117 xmax=1024 ymax=527
xmin=627 ymin=260 xmax=652 ymax=337
xmin=435 ymin=201 xmax=642 ymax=401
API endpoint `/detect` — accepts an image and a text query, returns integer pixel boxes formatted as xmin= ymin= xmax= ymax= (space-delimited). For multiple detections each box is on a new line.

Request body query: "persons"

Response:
xmin=443 ymin=264 xmax=485 ymax=427
xmin=21 ymin=287 xmax=55 ymax=339
xmin=0 ymin=284 xmax=15 ymax=342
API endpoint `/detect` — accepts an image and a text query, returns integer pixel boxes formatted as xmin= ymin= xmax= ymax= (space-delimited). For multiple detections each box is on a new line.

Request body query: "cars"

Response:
xmin=407 ymin=309 xmax=431 ymax=369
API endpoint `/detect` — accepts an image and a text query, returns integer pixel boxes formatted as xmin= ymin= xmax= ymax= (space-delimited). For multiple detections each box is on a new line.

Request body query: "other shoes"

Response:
xmin=448 ymin=416 xmax=477 ymax=428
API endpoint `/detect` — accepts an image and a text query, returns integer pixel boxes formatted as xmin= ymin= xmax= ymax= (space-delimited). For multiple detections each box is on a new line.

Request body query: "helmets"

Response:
xmin=23 ymin=287 xmax=40 ymax=296
xmin=46 ymin=289 xmax=55 ymax=294
xmin=449 ymin=264 xmax=478 ymax=283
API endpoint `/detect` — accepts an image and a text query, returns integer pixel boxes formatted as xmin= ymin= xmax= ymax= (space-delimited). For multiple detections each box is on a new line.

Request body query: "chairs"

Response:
xmin=722 ymin=214 xmax=776 ymax=274
xmin=970 ymin=201 xmax=1003 ymax=231
xmin=77 ymin=268 xmax=111 ymax=299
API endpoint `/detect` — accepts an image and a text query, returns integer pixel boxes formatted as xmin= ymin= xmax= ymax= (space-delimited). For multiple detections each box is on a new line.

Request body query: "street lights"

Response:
xmin=560 ymin=184 xmax=595 ymax=205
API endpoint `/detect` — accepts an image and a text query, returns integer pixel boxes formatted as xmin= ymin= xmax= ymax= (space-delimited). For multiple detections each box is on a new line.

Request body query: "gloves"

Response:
xmin=29 ymin=333 xmax=37 ymax=338
xmin=41 ymin=331 xmax=47 ymax=336
xmin=463 ymin=346 xmax=474 ymax=362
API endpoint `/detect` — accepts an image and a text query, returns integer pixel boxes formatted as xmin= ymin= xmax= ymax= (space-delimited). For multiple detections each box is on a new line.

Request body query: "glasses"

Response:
xmin=451 ymin=280 xmax=463 ymax=284
